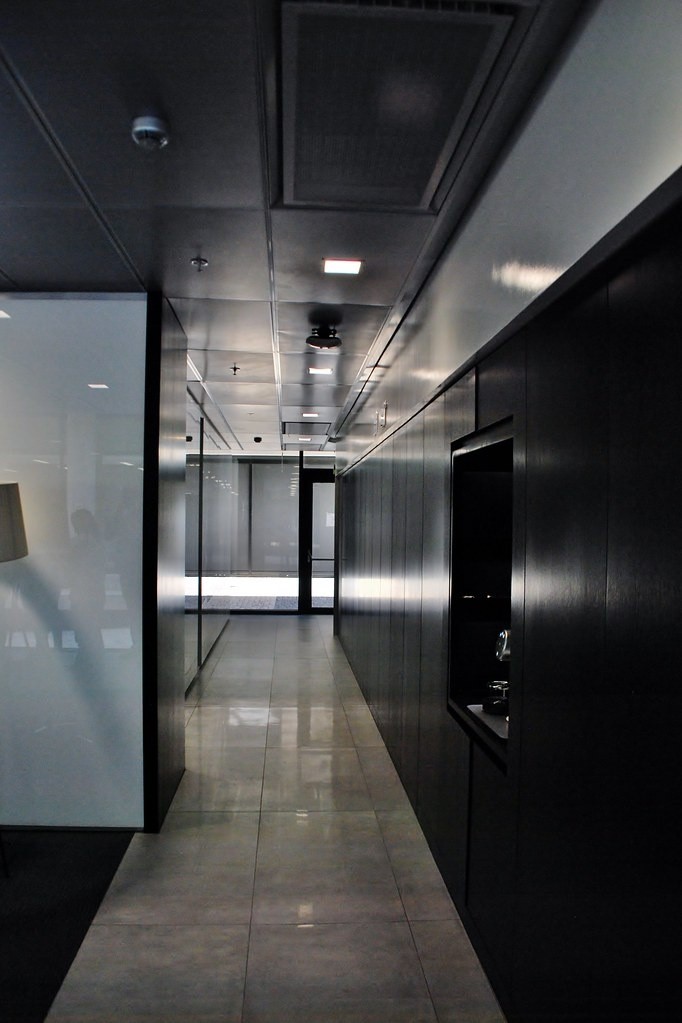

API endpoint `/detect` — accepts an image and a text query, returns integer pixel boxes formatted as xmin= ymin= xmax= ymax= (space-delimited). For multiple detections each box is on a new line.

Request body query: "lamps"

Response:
xmin=0 ymin=481 xmax=29 ymax=562
